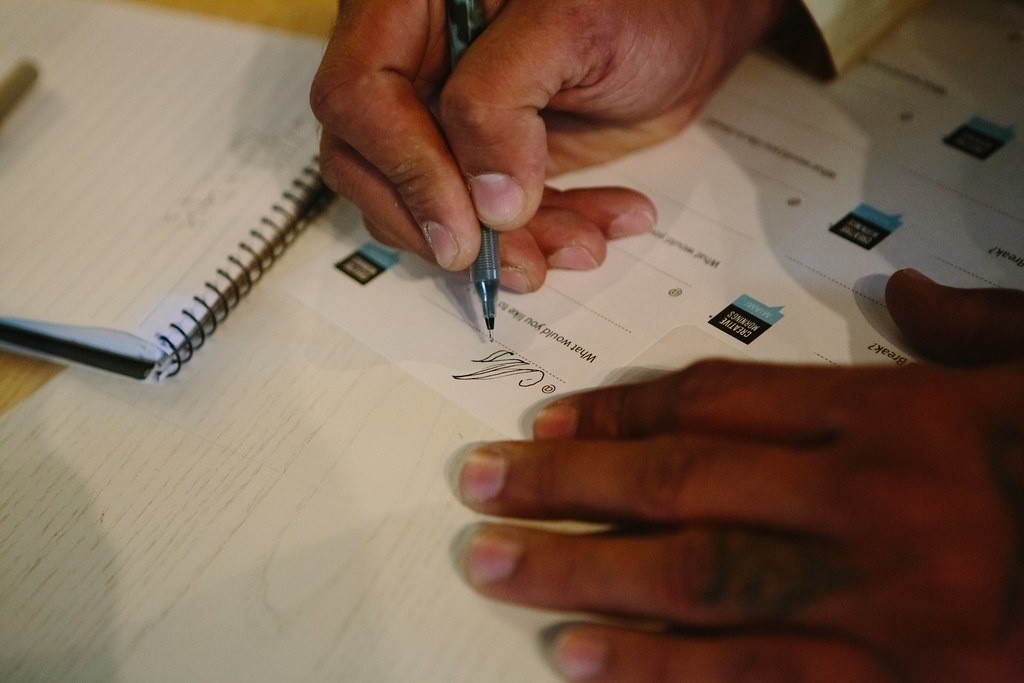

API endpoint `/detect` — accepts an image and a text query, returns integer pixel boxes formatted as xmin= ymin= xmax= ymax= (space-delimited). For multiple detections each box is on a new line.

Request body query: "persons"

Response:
xmin=309 ymin=0 xmax=1024 ymax=683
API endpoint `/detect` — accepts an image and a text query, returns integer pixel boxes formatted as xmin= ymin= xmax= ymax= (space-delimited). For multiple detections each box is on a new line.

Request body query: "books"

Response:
xmin=0 ymin=0 xmax=330 ymax=385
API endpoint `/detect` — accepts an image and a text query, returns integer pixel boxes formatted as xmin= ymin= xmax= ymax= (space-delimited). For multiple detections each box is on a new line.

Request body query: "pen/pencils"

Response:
xmin=443 ymin=1 xmax=502 ymax=345
